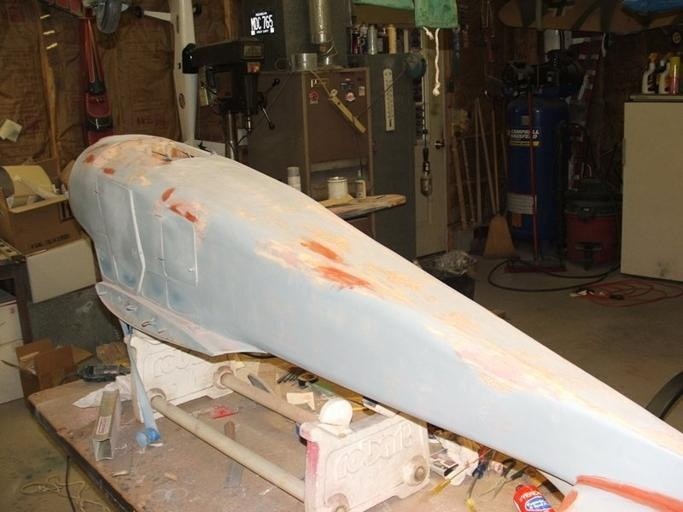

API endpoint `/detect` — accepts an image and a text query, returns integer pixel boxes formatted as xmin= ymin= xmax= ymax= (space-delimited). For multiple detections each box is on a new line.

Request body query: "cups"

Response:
xmin=345 ymin=26 xmax=352 ymax=54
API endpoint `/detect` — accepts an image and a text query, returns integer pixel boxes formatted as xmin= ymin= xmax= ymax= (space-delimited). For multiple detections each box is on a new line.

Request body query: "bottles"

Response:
xmin=511 ymin=485 xmax=557 ymax=512
xmin=354 ymin=175 xmax=366 ymax=200
xmin=670 ymin=56 xmax=680 ymax=95
xmin=387 ymin=24 xmax=396 ymax=54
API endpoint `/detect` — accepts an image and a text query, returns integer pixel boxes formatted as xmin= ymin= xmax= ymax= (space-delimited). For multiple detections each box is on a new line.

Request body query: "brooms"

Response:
xmin=483 ymin=105 xmax=518 ymax=258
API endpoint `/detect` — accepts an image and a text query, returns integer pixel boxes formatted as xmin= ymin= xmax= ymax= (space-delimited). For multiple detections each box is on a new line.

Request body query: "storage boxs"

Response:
xmin=22 ymin=220 xmax=96 ymax=304
xmin=0 ymin=287 xmax=25 ymax=403
xmin=0 ymin=160 xmax=69 ymax=258
xmin=0 ymin=337 xmax=93 ymax=412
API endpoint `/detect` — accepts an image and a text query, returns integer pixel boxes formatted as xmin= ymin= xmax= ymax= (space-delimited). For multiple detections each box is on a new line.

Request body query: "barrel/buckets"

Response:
xmin=563 ymin=208 xmax=614 ymax=268
xmin=506 ymin=91 xmax=560 ymax=246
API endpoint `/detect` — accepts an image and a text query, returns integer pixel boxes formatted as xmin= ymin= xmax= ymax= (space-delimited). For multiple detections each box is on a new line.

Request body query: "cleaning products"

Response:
xmin=658 ymin=54 xmax=674 ymax=95
xmin=642 ymin=54 xmax=657 ymax=94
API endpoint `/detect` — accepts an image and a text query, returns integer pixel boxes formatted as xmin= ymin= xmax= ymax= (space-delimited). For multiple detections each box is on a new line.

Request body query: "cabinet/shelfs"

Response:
xmin=244 ymin=67 xmax=378 ymax=240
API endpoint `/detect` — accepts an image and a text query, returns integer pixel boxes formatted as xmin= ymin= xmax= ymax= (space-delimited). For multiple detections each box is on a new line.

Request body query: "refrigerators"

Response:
xmin=622 ymin=94 xmax=683 ymax=284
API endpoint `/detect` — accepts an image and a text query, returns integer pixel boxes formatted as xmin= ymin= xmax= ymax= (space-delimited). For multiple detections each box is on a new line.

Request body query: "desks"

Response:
xmin=314 ymin=192 xmax=407 ymax=237
xmin=0 ymin=238 xmax=34 ymax=345
